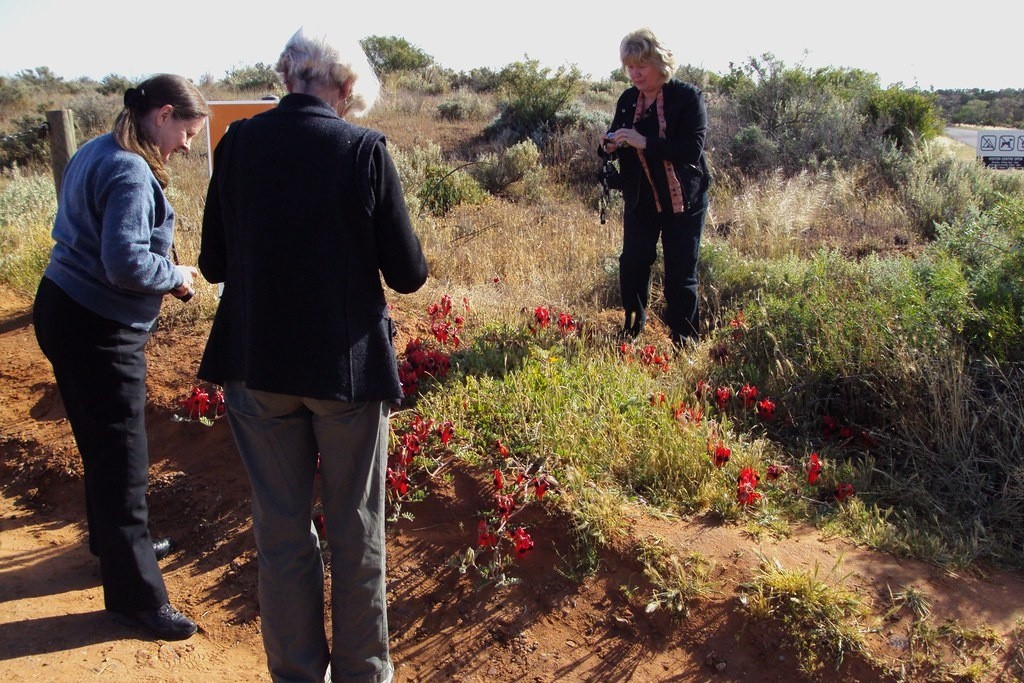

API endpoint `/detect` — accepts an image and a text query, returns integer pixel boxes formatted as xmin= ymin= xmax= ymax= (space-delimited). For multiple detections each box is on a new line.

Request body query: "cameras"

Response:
xmin=169 ymin=288 xmax=195 ymax=303
xmin=607 ymin=133 xmax=622 ymax=147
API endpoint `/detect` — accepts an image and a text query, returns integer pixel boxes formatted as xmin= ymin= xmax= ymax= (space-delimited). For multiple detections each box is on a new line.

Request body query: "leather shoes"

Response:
xmin=111 ymin=602 xmax=197 ymax=641
xmin=153 ymin=537 xmax=172 ymax=560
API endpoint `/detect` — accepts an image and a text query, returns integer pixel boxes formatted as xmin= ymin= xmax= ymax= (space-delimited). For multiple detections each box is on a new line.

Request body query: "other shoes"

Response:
xmin=383 ymin=662 xmax=394 ymax=682
xmin=618 ymin=326 xmax=643 ymax=342
xmin=323 ymin=665 xmax=332 ymax=683
xmin=674 ymin=336 xmax=700 ymax=357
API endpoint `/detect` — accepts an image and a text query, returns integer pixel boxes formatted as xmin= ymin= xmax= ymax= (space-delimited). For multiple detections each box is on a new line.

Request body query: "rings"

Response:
xmin=624 ymin=138 xmax=627 ymax=142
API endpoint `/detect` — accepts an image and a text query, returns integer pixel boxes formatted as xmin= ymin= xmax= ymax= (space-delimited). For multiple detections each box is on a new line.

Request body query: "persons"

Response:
xmin=33 ymin=73 xmax=209 ymax=641
xmin=596 ymin=27 xmax=714 ymax=365
xmin=196 ymin=24 xmax=430 ymax=683
